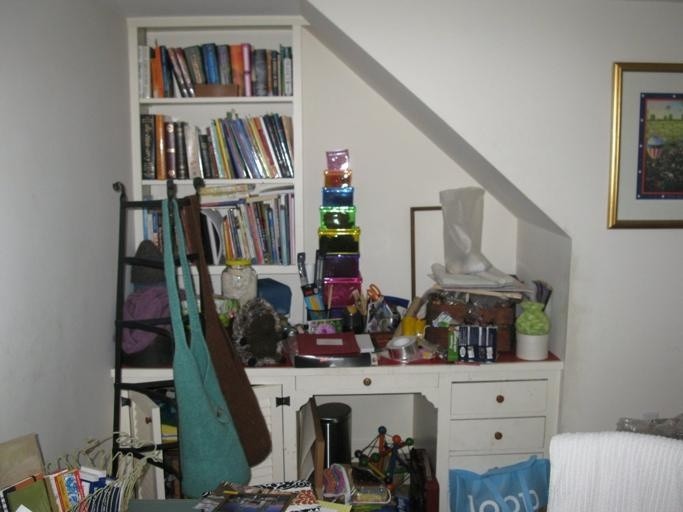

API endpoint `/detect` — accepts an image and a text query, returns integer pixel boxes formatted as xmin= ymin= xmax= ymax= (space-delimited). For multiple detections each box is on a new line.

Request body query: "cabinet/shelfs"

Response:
xmin=126 ymin=15 xmax=304 ymax=273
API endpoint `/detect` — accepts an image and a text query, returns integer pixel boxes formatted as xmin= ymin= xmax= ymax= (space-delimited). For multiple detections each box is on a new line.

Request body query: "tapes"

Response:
xmin=386 ymin=335 xmax=418 ymax=359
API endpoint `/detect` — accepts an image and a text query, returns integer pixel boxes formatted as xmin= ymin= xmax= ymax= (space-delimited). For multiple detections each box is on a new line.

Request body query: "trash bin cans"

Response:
xmin=317 ymin=403 xmax=351 ymax=468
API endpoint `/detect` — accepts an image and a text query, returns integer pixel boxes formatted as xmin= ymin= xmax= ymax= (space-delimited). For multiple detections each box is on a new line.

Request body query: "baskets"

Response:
xmin=46 ymin=432 xmax=157 ymax=512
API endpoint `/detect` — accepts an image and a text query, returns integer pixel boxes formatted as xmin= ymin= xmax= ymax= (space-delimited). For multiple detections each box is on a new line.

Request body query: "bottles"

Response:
xmin=220 ymin=257 xmax=258 ymax=304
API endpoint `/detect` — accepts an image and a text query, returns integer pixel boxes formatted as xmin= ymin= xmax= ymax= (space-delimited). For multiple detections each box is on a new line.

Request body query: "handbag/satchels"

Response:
xmin=206 ymin=320 xmax=271 ymax=467
xmin=449 ymin=455 xmax=550 ymax=512
xmin=173 ymin=335 xmax=250 ymax=498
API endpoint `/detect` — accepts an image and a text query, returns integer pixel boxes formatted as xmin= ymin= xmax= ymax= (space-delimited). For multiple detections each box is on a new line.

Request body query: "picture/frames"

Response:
xmin=606 ymin=61 xmax=683 ymax=229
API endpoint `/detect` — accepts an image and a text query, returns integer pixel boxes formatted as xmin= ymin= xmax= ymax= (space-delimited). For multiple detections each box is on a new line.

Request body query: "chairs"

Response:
xmin=545 ymin=429 xmax=682 ymax=512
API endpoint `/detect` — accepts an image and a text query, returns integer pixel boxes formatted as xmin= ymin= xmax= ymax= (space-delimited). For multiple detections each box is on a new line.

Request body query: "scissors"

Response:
xmin=367 ymin=284 xmax=380 ymax=301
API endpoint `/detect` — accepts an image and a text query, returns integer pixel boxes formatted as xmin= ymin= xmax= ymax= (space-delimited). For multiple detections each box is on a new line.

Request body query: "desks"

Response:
xmin=113 ymin=329 xmax=564 ymax=511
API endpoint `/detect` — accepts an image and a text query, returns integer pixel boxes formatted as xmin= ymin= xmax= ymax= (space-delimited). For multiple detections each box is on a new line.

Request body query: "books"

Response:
xmin=0 ymin=463 xmax=124 ymax=512
xmin=133 ymin=39 xmax=293 ymax=265
